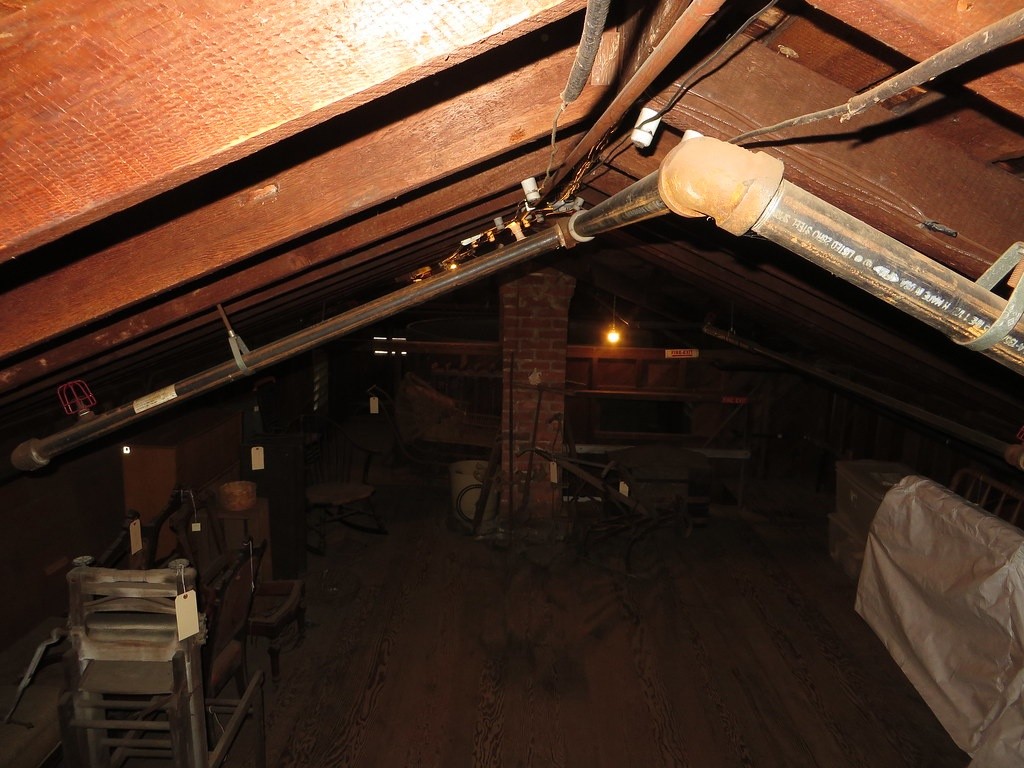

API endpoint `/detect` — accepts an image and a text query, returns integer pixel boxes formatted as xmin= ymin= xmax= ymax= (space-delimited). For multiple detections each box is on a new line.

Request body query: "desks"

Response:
xmin=575 ymin=443 xmax=752 ymax=510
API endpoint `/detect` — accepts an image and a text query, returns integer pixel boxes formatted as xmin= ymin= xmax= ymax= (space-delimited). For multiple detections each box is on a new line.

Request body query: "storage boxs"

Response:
xmin=826 ymin=511 xmax=867 ymax=593
xmin=837 ymin=459 xmax=920 ymax=538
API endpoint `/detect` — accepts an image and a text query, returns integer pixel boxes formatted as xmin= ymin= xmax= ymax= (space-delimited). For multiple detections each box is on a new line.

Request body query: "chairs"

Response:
xmin=54 ymin=490 xmax=304 ymax=768
xmin=283 ymin=414 xmax=390 ymax=553
